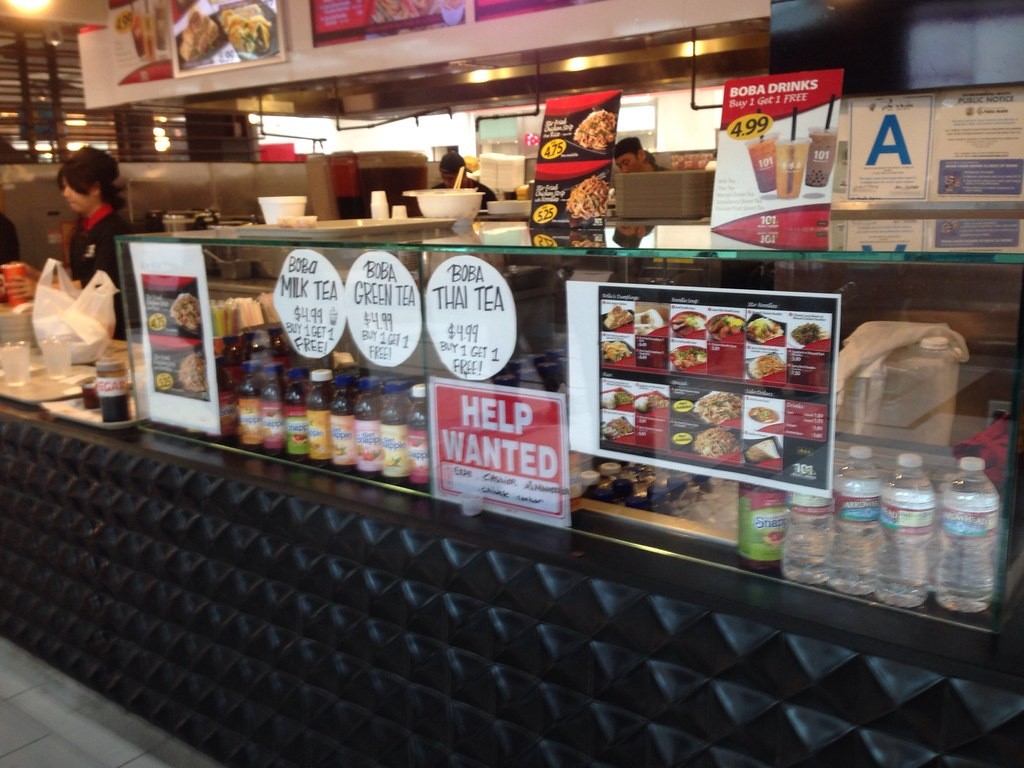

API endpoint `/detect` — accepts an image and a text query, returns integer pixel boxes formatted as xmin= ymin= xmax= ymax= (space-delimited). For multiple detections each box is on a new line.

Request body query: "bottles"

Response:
xmin=738 ymin=445 xmax=1000 ymax=613
xmin=215 ymin=326 xmax=429 ymax=491
xmin=580 ymin=462 xmax=716 ymax=516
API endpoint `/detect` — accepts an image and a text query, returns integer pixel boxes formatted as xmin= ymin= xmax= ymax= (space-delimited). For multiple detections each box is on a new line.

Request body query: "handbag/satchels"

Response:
xmin=32 ymin=258 xmax=120 ymax=364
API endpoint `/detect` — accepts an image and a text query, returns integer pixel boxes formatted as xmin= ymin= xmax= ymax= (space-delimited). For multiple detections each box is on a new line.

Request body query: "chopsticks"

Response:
xmin=453 ymin=166 xmax=465 ymax=189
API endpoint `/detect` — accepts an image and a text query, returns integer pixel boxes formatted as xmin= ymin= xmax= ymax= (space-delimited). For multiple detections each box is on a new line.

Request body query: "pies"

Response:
xmin=605 ymin=304 xmax=633 ymax=328
xmin=178 ymin=10 xmax=271 ymax=61
xmin=601 ymin=391 xmax=657 ymax=412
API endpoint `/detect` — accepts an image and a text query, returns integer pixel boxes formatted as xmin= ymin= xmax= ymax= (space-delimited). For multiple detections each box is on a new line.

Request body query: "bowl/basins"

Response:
xmin=258 ymin=196 xmax=307 ymax=225
xmin=416 ymin=192 xmax=486 ymax=228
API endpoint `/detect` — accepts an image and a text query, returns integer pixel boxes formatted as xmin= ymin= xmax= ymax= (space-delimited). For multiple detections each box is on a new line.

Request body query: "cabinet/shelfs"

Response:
xmin=115 ymin=233 xmax=1024 ymax=635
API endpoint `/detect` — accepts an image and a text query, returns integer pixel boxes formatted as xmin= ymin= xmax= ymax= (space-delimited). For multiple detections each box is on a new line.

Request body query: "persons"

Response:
xmin=432 ymin=150 xmax=498 ymax=210
xmin=614 ymin=136 xmax=671 ymax=172
xmin=611 ymin=222 xmax=655 ymax=249
xmin=486 ymin=258 xmax=566 ymax=397
xmin=57 ymin=146 xmax=141 ymax=342
xmin=0 ymin=210 xmax=20 ymax=265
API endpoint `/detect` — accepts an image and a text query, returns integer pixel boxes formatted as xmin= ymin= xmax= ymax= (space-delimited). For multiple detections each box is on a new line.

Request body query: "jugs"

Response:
xmin=836 ymin=337 xmax=960 ymax=447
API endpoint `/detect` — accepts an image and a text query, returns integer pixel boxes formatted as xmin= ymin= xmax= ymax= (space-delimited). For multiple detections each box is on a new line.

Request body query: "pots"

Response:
xmin=161 ymin=209 xmax=219 ymax=232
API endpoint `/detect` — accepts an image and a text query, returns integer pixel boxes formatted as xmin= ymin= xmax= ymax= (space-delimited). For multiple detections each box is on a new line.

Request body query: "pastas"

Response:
xmin=178 ymin=352 xmax=207 ymax=393
xmin=568 ymin=174 xmax=609 ymax=219
xmin=573 ymin=109 xmax=616 ymax=151
xmin=172 ymin=293 xmax=200 ymax=330
xmin=692 ymin=427 xmax=740 ymax=457
xmin=697 ymin=390 xmax=742 ymax=425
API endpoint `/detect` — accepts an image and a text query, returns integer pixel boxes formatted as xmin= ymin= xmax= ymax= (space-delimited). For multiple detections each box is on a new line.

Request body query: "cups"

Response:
xmin=371 ymin=191 xmax=408 ymax=219
xmin=0 ymin=263 xmax=27 ymax=307
xmin=42 ymin=335 xmax=72 ymax=380
xmin=0 ymin=341 xmax=31 ymax=387
xmin=745 ymin=127 xmax=838 ymax=200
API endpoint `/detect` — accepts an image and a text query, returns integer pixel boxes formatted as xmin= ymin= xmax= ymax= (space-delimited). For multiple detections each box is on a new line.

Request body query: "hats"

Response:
xmin=613 ymin=137 xmax=643 ymax=159
xmin=438 ymin=153 xmax=473 ymax=173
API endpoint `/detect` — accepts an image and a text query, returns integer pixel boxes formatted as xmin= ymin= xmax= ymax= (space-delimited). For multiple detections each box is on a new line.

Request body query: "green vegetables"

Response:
xmin=792 ymin=322 xmax=822 ymax=345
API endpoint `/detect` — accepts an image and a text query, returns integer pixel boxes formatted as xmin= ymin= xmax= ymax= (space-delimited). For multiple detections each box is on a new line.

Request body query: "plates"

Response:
xmin=487 ymin=200 xmax=532 ymax=214
xmin=0 ymin=366 xmax=97 ymax=405
xmin=40 ymin=396 xmax=138 ymax=430
xmin=601 ymin=310 xmax=828 ymax=462
